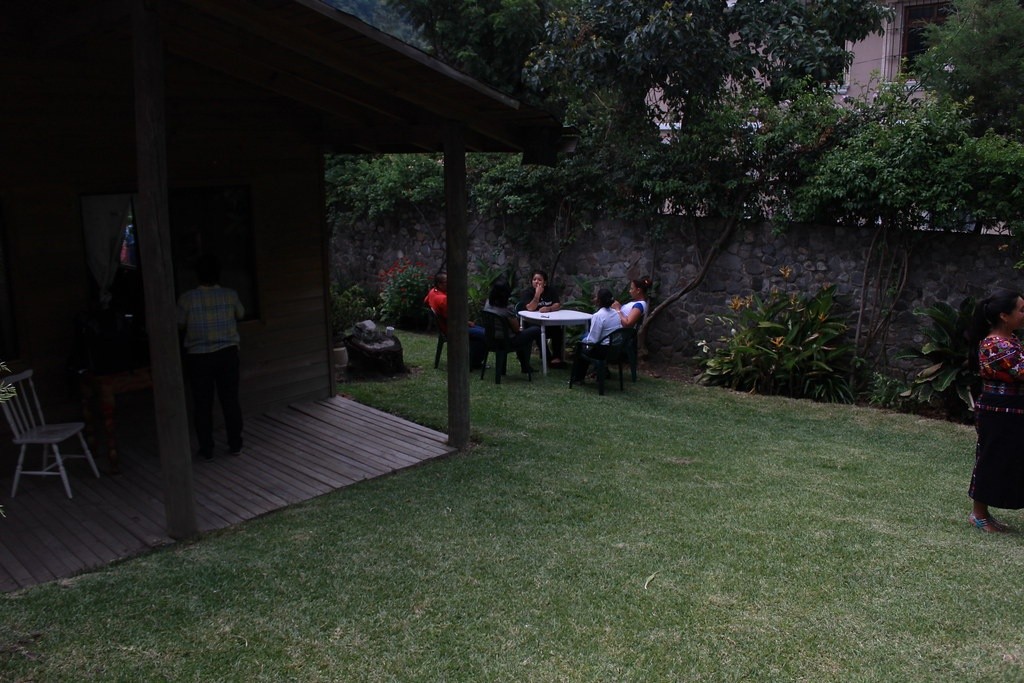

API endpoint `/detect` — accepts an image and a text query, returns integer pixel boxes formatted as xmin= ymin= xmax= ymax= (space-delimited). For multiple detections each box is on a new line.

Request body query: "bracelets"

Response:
xmin=617 ymin=311 xmax=621 ymax=313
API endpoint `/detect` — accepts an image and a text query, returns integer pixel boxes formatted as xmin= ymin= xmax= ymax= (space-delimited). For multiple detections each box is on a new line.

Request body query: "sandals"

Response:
xmin=970 ymin=512 xmax=1009 ymax=533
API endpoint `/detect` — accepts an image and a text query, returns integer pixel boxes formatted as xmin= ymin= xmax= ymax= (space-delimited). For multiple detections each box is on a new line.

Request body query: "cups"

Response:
xmin=386 ymin=325 xmax=394 ymax=337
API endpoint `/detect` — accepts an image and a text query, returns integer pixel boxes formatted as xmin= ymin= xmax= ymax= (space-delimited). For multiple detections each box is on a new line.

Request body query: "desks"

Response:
xmin=518 ymin=309 xmax=594 ymax=378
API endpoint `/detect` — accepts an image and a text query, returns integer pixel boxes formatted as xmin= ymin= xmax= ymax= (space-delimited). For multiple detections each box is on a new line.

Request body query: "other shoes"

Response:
xmin=474 ymin=362 xmax=491 ymax=369
xmin=567 ymin=378 xmax=584 ymax=388
xmin=548 ymin=357 xmax=561 ymax=367
xmin=521 ymin=368 xmax=539 ymax=374
xmin=198 ymin=448 xmax=215 ymax=462
xmin=584 ymin=372 xmax=597 ymax=384
xmin=230 ymin=442 xmax=246 ymax=455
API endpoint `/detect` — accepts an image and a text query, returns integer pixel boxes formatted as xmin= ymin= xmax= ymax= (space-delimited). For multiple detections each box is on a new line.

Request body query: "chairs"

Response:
xmin=479 ymin=310 xmax=531 ymax=385
xmin=430 ymin=307 xmax=450 ymax=370
xmin=0 ymin=368 xmax=102 ymax=500
xmin=568 ymin=327 xmax=634 ymax=396
xmin=604 ymin=313 xmax=646 ymax=383
xmin=515 ymin=299 xmax=551 ymax=360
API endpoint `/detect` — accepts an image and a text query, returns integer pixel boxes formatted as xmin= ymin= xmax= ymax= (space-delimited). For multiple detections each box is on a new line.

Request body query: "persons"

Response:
xmin=482 ymin=275 xmax=552 ymax=376
xmin=176 ymin=254 xmax=243 ymax=462
xmin=423 ymin=269 xmax=491 ymax=373
xmin=583 ymin=277 xmax=652 ymax=385
xmin=521 ymin=271 xmax=568 ymax=368
xmin=968 ymin=290 xmax=1024 ymax=533
xmin=564 ymin=288 xmax=624 ymax=385
